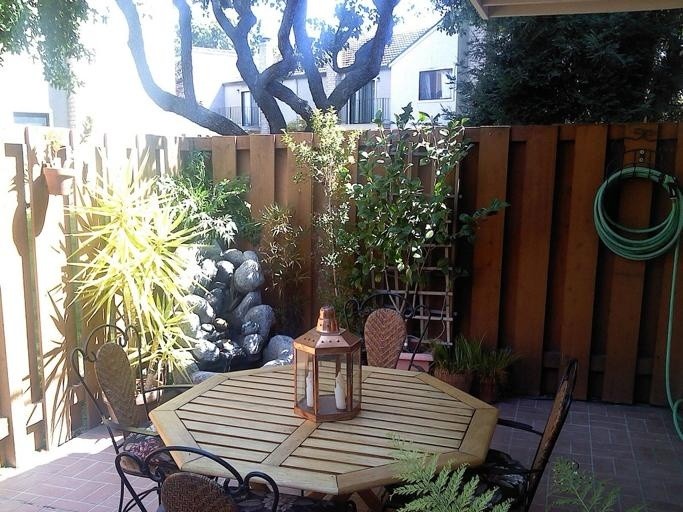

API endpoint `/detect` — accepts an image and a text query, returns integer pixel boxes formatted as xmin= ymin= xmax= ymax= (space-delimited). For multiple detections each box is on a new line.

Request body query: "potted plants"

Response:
xmin=478 ymin=347 xmax=519 ymax=404
xmin=333 ymin=100 xmax=508 ymax=372
xmin=42 ymin=132 xmax=75 ymax=195
xmin=434 ymin=338 xmax=476 ymax=394
xmin=62 ymin=148 xmax=211 ymax=434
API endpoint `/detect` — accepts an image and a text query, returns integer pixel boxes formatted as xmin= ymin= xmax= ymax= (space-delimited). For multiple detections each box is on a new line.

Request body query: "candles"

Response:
xmin=306 ymin=371 xmax=314 ymax=384
xmin=335 ymin=371 xmax=347 ymax=397
xmin=307 ymin=379 xmax=314 ymax=407
xmin=334 ymin=382 xmax=346 ymax=410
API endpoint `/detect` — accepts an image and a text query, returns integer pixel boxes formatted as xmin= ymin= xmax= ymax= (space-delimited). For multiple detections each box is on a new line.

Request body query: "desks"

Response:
xmin=148 ymin=361 xmax=500 ymax=511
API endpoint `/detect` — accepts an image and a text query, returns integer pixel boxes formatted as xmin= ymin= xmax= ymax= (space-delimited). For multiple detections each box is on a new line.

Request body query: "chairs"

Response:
xmin=343 ymin=293 xmax=431 ymax=371
xmin=70 ymin=325 xmax=197 ymax=512
xmin=115 ymin=446 xmax=279 ymax=512
xmin=385 ymin=357 xmax=581 ymax=511
